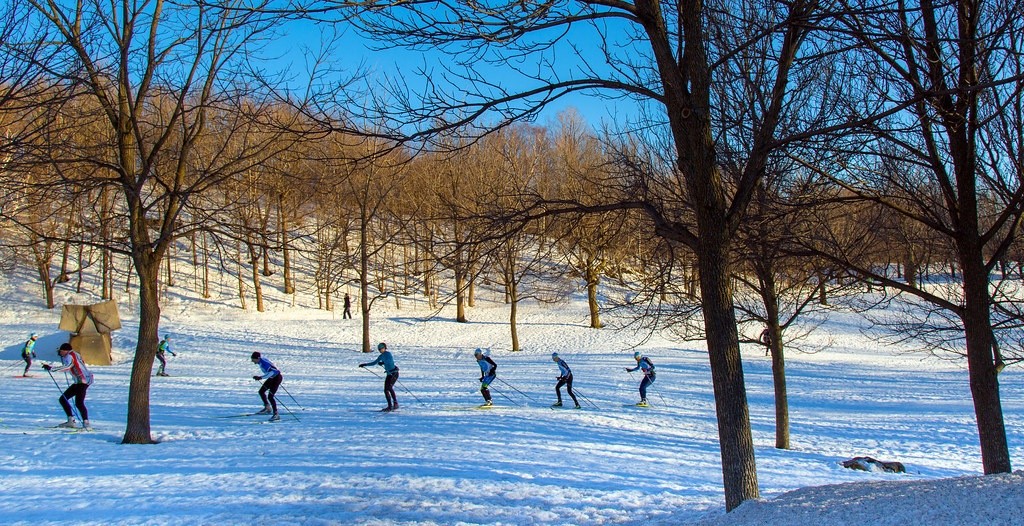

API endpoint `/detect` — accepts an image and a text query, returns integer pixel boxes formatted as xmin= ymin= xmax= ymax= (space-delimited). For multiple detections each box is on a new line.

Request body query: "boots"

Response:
xmin=636 ymin=399 xmax=646 ymax=405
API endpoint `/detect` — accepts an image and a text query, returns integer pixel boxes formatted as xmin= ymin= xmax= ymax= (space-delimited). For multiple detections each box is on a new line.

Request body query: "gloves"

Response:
xmin=642 ymin=368 xmax=646 ymax=372
xmin=489 ymin=372 xmax=493 ymax=377
xmin=25 ymin=354 xmax=28 ymax=358
xmin=556 ymin=377 xmax=561 ymax=381
xmin=32 ymin=353 xmax=36 ymax=358
xmin=158 ymin=353 xmax=161 ymax=357
xmin=253 ymin=376 xmax=262 ymax=381
xmin=378 ymin=361 xmax=385 ymax=366
xmin=42 ymin=365 xmax=52 ymax=370
xmin=58 ymin=350 xmax=63 ymax=357
xmin=479 ymin=376 xmax=484 ymax=380
xmin=359 ymin=363 xmax=367 ymax=367
xmin=561 ymin=377 xmax=566 ymax=381
xmin=173 ymin=354 xmax=176 ymax=356
xmin=627 ymin=369 xmax=632 ymax=372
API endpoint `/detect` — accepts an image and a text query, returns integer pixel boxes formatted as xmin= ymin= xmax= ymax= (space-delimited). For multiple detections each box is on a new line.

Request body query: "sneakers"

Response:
xmin=484 ymin=400 xmax=492 ymax=406
xmin=575 ymin=405 xmax=581 ymax=409
xmin=269 ymin=415 xmax=280 ymax=421
xmin=59 ymin=417 xmax=76 ymax=427
xmin=83 ymin=420 xmax=92 ymax=431
xmin=554 ymin=402 xmax=562 ymax=406
xmin=259 ymin=408 xmax=271 ymax=414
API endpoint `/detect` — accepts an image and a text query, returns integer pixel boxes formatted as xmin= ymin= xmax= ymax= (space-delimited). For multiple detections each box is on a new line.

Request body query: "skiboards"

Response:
xmin=10 ymin=375 xmax=39 ymax=379
xmin=220 ymin=410 xmax=299 ymax=426
xmin=42 ymin=424 xmax=98 ymax=436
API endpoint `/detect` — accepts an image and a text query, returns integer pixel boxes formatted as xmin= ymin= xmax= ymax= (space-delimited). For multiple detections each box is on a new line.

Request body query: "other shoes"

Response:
xmin=157 ymin=372 xmax=169 ymax=376
xmin=392 ymin=404 xmax=399 ymax=409
xmin=382 ymin=407 xmax=392 ymax=410
xmin=24 ymin=372 xmax=31 ymax=377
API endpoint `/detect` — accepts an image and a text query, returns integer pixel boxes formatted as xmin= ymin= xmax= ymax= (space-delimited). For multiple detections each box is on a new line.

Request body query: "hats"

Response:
xmin=378 ymin=343 xmax=386 ymax=349
xmin=60 ymin=343 xmax=72 ymax=350
xmin=634 ymin=352 xmax=640 ymax=358
xmin=165 ymin=334 xmax=171 ymax=339
xmin=475 ymin=348 xmax=481 ymax=354
xmin=31 ymin=333 xmax=37 ymax=338
xmin=552 ymin=353 xmax=558 ymax=358
xmin=251 ymin=352 xmax=260 ymax=359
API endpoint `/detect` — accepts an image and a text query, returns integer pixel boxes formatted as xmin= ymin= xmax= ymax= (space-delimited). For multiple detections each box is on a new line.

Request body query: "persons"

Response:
xmin=41 ymin=342 xmax=93 ymax=427
xmin=626 ymin=351 xmax=656 ymax=406
xmin=155 ymin=334 xmax=176 ymax=377
xmin=343 ymin=293 xmax=351 ymax=319
xmin=552 ymin=352 xmax=582 ymax=409
xmin=250 ymin=352 xmax=283 ymax=422
xmin=21 ymin=332 xmax=38 ymax=377
xmin=359 ymin=342 xmax=399 ymax=412
xmin=474 ymin=348 xmax=497 ymax=407
xmin=760 ymin=325 xmax=771 ymax=356
